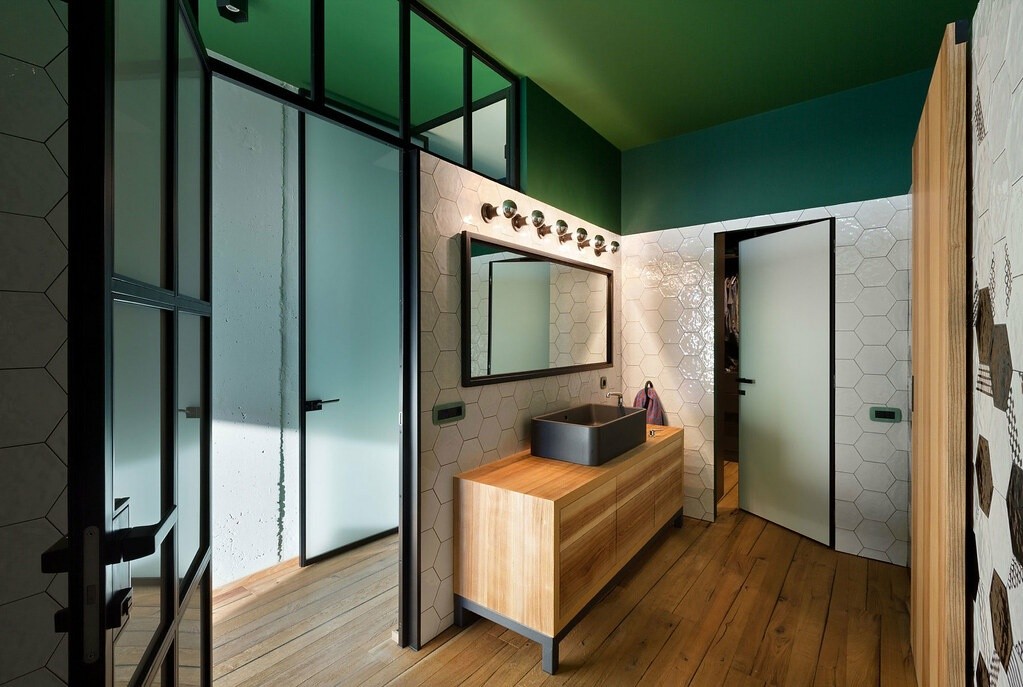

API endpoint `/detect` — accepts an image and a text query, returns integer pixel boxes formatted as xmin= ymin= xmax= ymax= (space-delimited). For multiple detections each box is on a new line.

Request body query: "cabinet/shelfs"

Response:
xmin=453 ymin=424 xmax=685 ymax=675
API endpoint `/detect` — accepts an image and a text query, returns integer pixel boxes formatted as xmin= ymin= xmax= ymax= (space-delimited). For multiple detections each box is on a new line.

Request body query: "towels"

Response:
xmin=633 ymin=388 xmax=664 ymax=426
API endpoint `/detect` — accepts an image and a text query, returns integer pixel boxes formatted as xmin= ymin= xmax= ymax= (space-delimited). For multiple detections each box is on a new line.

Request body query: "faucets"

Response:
xmin=605 ymin=392 xmax=623 ymax=406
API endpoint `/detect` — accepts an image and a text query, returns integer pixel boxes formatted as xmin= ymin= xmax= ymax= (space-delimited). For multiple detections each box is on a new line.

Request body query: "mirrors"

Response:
xmin=461 ymin=230 xmax=614 ymax=388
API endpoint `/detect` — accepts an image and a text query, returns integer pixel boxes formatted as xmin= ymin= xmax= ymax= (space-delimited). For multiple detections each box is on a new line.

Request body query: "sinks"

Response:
xmin=531 ymin=402 xmax=647 ymax=466
